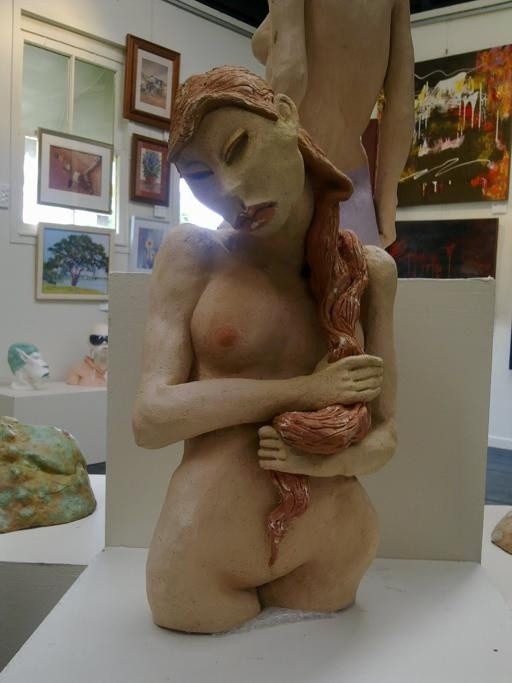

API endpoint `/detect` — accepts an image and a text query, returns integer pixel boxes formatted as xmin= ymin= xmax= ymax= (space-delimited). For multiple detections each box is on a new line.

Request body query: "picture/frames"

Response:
xmin=130 ymin=133 xmax=171 ymax=208
xmin=36 ymin=127 xmax=114 ymax=214
xmin=124 ymin=33 xmax=182 ymax=131
xmin=36 ymin=223 xmax=116 ymax=302
xmin=129 ymin=215 xmax=170 ymax=273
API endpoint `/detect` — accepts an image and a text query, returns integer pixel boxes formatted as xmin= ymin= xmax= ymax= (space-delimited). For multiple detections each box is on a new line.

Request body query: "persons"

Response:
xmin=128 ymin=68 xmax=399 ymax=636
xmin=256 ymin=0 xmax=418 ymax=251
